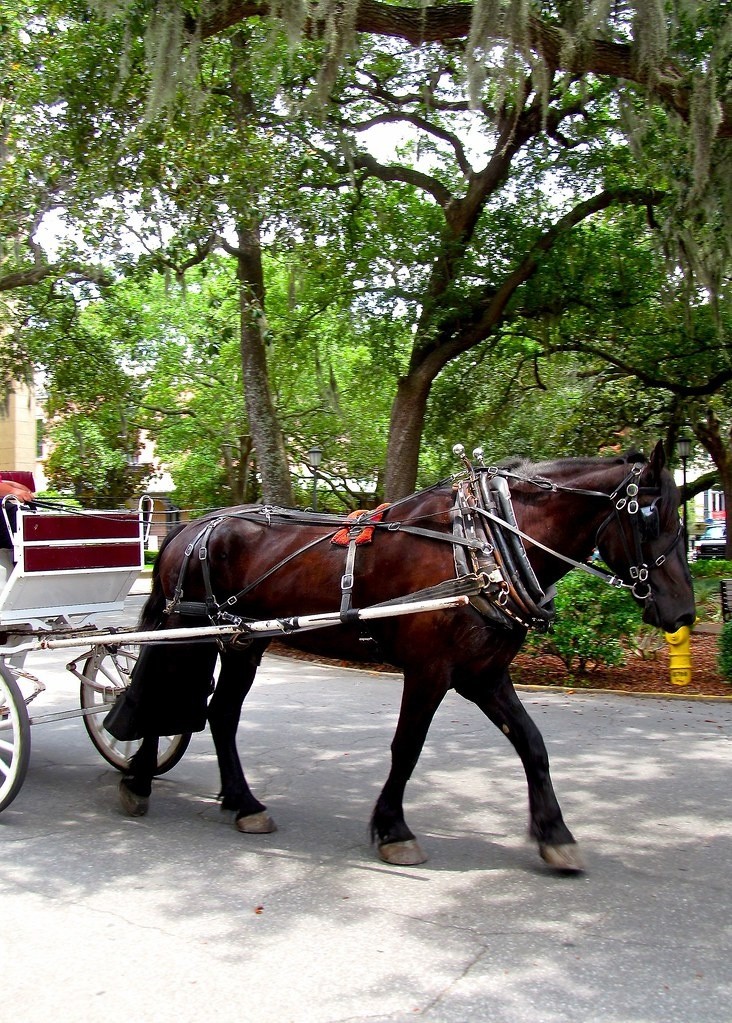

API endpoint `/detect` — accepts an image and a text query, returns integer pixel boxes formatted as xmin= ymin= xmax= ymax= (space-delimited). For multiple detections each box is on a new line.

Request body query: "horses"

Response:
xmin=100 ymin=437 xmax=697 ymax=874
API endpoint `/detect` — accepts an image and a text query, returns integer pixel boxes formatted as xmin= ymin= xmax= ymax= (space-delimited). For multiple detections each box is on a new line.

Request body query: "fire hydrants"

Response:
xmin=665 ymin=625 xmax=693 ymax=687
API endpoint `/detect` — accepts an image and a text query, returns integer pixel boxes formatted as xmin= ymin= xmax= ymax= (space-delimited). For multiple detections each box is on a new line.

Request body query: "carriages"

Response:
xmin=0 ymin=430 xmax=699 ymax=873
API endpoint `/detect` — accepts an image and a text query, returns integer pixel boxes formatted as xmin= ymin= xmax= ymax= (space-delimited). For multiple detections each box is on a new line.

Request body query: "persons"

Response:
xmin=0 ymin=474 xmax=37 ymax=567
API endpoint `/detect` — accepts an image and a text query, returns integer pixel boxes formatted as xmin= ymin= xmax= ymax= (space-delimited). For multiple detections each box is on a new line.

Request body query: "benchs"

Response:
xmin=0 ymin=472 xmax=37 ymax=522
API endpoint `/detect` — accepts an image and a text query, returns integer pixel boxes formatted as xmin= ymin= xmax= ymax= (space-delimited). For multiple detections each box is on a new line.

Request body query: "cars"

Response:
xmin=692 ymin=525 xmax=728 ymax=562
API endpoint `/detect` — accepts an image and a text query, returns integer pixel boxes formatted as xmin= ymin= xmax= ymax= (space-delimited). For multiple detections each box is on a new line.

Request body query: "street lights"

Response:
xmin=673 ymin=430 xmax=692 ymax=554
xmin=309 ymin=443 xmax=322 ymax=510
